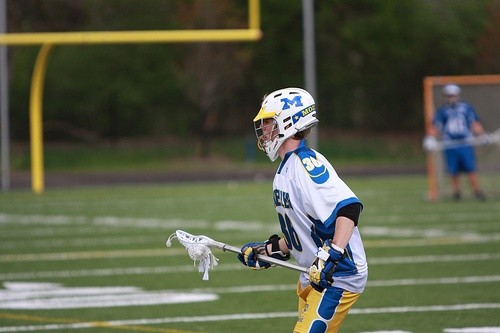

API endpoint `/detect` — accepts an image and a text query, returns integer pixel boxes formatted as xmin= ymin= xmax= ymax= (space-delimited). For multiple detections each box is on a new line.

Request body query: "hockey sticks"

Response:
xmin=419 ymin=131 xmax=500 ymax=150
xmin=166 ymin=229 xmax=310 ymax=281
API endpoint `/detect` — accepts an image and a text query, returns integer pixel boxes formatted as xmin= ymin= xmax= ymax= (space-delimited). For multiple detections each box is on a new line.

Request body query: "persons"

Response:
xmin=421 ymin=83 xmax=490 ymax=203
xmin=238 ymin=87 xmax=370 ymax=333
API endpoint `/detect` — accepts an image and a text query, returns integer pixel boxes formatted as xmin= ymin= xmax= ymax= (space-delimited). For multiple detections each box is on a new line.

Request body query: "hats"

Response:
xmin=444 ymin=84 xmax=461 ymax=96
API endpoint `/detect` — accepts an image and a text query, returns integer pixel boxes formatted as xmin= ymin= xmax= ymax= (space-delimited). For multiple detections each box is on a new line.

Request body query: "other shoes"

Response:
xmin=476 ymin=190 xmax=485 ymax=200
xmin=454 ymin=192 xmax=461 ymax=201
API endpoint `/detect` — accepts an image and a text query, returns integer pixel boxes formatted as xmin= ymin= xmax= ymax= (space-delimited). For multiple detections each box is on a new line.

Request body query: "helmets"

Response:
xmin=252 ymin=87 xmax=319 ymax=161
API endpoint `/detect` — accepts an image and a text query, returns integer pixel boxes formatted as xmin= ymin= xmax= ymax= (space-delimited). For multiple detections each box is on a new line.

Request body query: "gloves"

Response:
xmin=424 ymin=136 xmax=435 ymax=150
xmin=236 ymin=234 xmax=290 ymax=271
xmin=308 ymin=237 xmax=346 ymax=292
